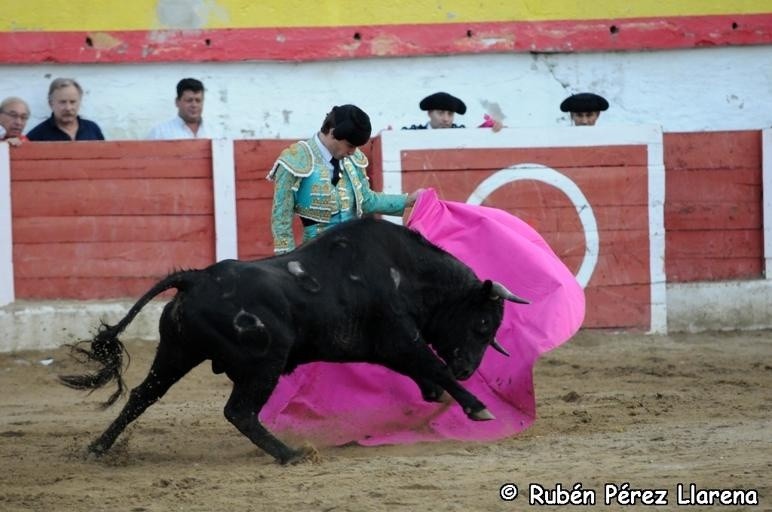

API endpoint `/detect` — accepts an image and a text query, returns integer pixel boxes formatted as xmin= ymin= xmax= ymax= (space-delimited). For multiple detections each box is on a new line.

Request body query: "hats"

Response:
xmin=560 ymin=91 xmax=609 ymax=113
xmin=419 ymin=91 xmax=467 ymax=116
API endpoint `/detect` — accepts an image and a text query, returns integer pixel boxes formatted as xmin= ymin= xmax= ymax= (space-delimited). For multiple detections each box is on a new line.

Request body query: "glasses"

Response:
xmin=1 ymin=109 xmax=29 ymax=122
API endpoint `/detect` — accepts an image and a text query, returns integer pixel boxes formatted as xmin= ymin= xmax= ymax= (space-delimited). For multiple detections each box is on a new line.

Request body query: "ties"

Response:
xmin=329 ymin=157 xmax=342 ymax=187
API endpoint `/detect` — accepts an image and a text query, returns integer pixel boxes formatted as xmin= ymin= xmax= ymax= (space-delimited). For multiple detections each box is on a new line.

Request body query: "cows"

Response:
xmin=55 ymin=216 xmax=532 ymax=469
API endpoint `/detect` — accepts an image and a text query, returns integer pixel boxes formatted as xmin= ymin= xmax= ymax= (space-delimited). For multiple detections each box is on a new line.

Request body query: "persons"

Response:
xmin=147 ymin=79 xmax=223 ymax=139
xmin=560 ymin=93 xmax=609 ymax=126
xmin=265 ymin=105 xmax=426 ymax=256
xmin=0 ymin=97 xmax=31 ymax=146
xmin=26 ymin=77 xmax=104 ymax=141
xmin=387 ymin=92 xmax=503 ymax=133
